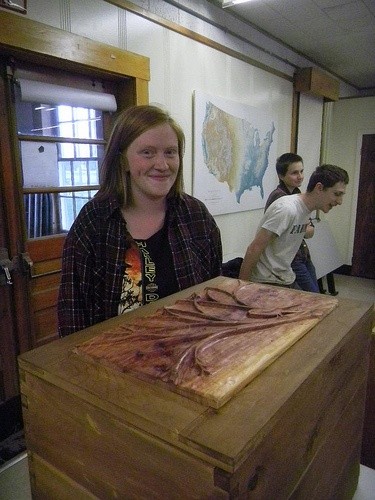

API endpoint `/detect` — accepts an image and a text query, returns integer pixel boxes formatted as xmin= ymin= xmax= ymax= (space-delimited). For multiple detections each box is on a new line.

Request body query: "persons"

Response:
xmin=238 ymin=165 xmax=349 ymax=288
xmin=265 ymin=153 xmax=319 ymax=294
xmin=57 ymin=104 xmax=222 ymax=338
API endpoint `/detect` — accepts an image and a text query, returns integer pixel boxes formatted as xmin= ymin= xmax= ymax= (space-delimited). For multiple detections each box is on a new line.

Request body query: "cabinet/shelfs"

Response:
xmin=0 ymin=12 xmax=150 ymax=406
xmin=17 ymin=275 xmax=374 ymax=500
